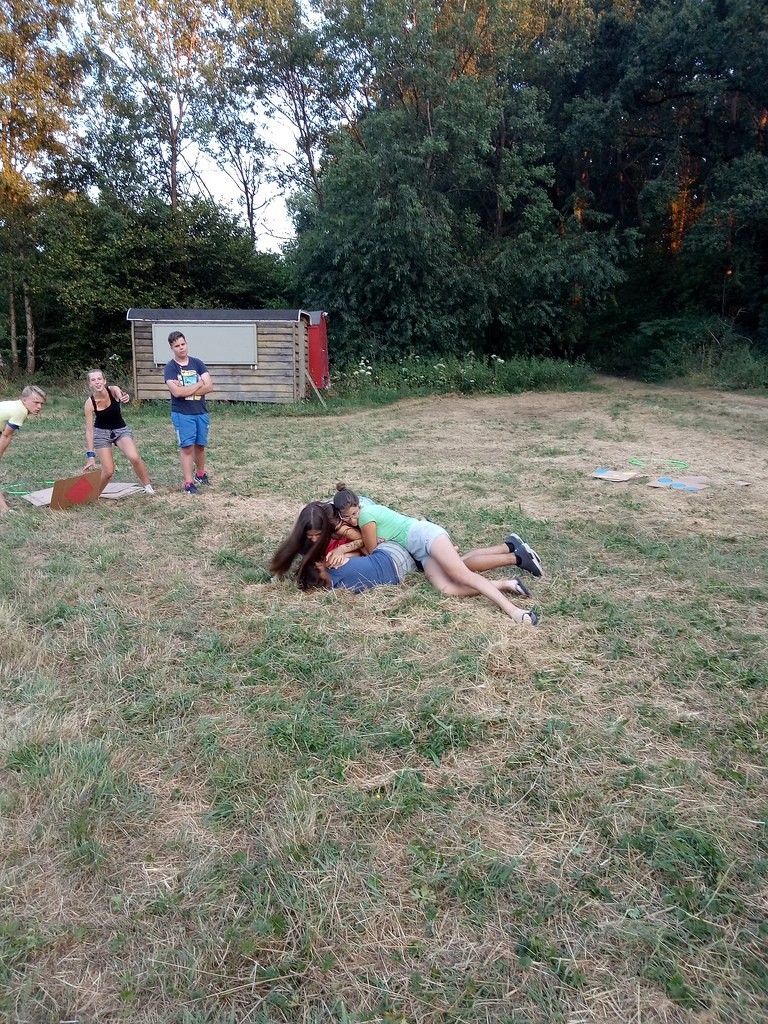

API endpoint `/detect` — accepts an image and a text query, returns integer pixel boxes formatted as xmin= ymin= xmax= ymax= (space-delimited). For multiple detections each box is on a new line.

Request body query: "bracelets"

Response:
xmin=86 ymin=452 xmax=95 ymax=457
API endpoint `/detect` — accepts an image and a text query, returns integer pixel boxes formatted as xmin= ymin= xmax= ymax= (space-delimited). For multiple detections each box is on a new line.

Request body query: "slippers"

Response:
xmin=516 ymin=609 xmax=537 ymax=625
xmin=514 ymin=576 xmax=530 ymax=596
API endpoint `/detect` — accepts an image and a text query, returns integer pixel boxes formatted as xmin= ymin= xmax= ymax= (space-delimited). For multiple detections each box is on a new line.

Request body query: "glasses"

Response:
xmin=338 ymin=506 xmax=360 ymax=521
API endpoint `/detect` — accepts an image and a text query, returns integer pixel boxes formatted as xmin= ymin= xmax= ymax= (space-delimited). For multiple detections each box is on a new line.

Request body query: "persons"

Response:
xmin=265 ymin=482 xmax=545 ymax=625
xmin=80 ymin=368 xmax=156 ymax=496
xmin=163 ymin=331 xmax=215 ymax=497
xmin=0 ymin=385 xmax=46 ymax=518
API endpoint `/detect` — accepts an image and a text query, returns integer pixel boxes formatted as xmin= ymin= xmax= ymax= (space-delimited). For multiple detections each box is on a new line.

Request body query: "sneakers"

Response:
xmin=184 ymin=483 xmax=205 ymax=496
xmin=515 ymin=543 xmax=543 ymax=576
xmin=194 ymin=472 xmax=209 ymax=486
xmin=504 ymin=533 xmax=541 ymax=564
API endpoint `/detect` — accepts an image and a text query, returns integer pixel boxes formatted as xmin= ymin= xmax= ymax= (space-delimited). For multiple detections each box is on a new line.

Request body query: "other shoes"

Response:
xmin=145 ymin=484 xmax=155 ymax=494
